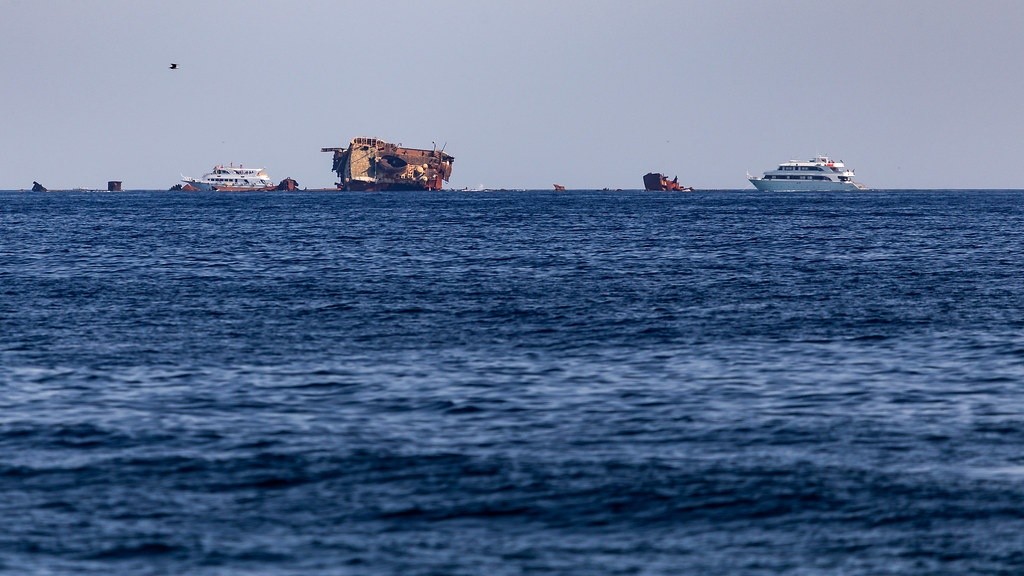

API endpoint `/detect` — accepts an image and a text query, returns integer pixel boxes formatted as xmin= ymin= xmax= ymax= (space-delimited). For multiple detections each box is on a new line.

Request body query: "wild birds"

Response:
xmin=169 ymin=64 xmax=179 ymax=69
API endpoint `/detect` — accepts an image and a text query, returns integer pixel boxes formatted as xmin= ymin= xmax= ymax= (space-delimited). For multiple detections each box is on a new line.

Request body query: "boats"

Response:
xmin=320 ymin=137 xmax=455 ymax=192
xmin=552 ymin=184 xmax=566 ymax=190
xmin=744 ymin=155 xmax=870 ymax=193
xmin=643 ymin=172 xmax=693 ymax=193
xmin=179 ymin=162 xmax=301 ymax=194
